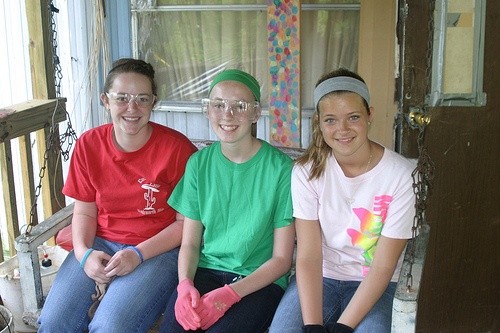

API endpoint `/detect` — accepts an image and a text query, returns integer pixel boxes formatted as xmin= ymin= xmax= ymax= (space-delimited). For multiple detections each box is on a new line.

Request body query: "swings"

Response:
xmin=14 ymin=0 xmax=431 ymax=333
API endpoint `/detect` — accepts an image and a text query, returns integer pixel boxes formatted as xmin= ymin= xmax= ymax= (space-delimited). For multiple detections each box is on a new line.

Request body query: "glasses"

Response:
xmin=104 ymin=92 xmax=157 ymax=107
xmin=201 ymin=98 xmax=261 ymax=126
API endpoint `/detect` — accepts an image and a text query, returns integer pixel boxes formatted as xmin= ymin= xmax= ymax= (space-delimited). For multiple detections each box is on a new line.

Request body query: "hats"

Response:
xmin=208 ymin=69 xmax=261 ymax=103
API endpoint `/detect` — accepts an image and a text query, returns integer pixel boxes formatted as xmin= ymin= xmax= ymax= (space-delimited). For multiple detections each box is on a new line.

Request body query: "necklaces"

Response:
xmin=329 ymin=141 xmax=373 ymax=207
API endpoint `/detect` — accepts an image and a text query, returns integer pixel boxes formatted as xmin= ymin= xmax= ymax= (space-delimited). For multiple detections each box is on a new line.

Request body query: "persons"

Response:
xmin=268 ymin=68 xmax=421 ymax=333
xmin=159 ymin=69 xmax=296 ymax=333
xmin=36 ymin=58 xmax=198 ymax=333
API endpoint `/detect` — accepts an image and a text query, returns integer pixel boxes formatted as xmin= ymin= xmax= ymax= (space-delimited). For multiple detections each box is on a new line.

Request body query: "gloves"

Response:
xmin=173 ymin=279 xmax=201 ymax=330
xmin=194 ymin=283 xmax=242 ymax=331
xmin=88 ymin=260 xmax=118 ymax=320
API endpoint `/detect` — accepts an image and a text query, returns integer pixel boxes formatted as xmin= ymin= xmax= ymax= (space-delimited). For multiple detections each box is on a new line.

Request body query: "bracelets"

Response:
xmin=126 ymin=246 xmax=143 ymax=264
xmin=80 ymin=247 xmax=94 ymax=271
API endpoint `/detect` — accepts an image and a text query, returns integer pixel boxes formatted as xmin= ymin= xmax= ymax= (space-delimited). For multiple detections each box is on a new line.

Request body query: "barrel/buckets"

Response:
xmin=0 ymin=245 xmax=68 ymax=332
xmin=0 ymin=305 xmax=15 ymax=333
